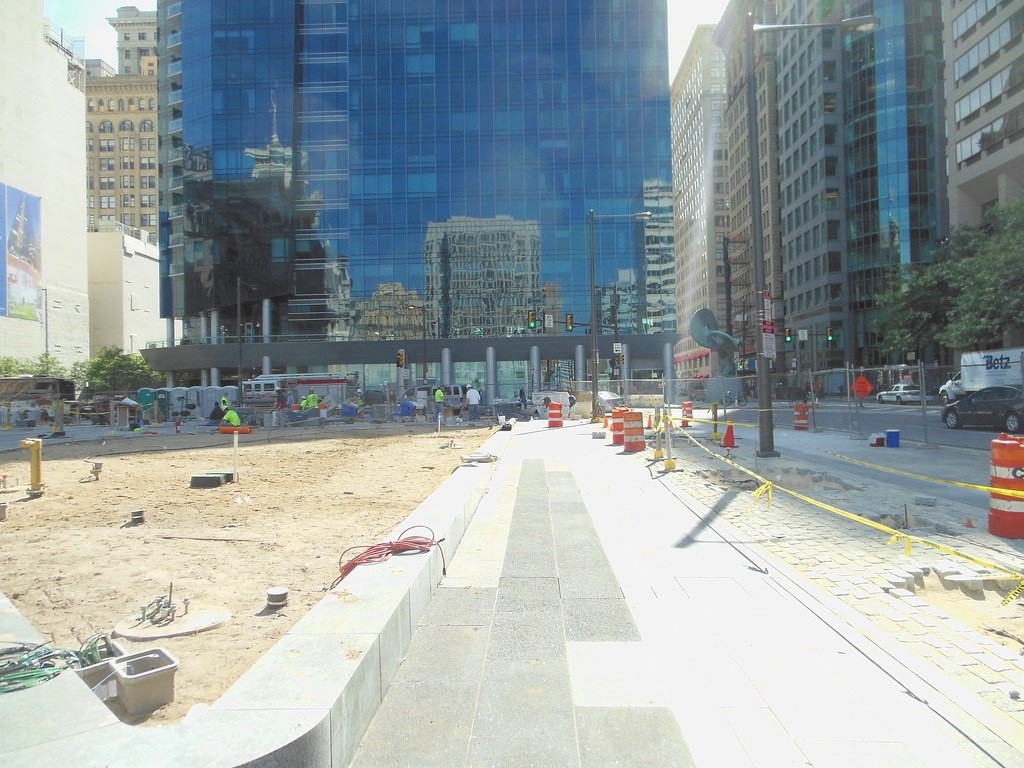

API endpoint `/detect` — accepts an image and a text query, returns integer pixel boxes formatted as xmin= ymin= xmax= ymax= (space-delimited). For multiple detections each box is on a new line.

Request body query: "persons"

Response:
xmin=275 ymin=387 xmax=320 ymax=412
xmin=432 ymin=386 xmax=446 ymax=422
xmin=464 ymin=383 xmax=481 ymax=422
xmin=210 ymin=392 xmax=242 ymax=427
xmin=353 ymin=388 xmax=365 ymax=408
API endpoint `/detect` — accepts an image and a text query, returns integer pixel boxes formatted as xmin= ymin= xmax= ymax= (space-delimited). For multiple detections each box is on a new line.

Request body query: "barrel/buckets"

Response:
xmin=886 ymin=429 xmax=900 ymax=446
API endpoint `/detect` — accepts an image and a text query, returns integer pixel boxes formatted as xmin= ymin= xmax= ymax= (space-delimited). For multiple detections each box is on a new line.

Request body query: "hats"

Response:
xmin=439 ymin=384 xmax=446 ymax=391
xmin=222 ymin=406 xmax=228 ymax=411
xmin=466 ymin=384 xmax=471 ymax=388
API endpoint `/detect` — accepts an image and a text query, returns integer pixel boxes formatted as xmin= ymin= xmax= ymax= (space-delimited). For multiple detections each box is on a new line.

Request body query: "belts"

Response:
xmin=436 ymin=402 xmax=443 ymax=403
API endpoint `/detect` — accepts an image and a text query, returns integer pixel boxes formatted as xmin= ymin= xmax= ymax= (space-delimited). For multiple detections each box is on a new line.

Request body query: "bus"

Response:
xmin=0 ymin=374 xmax=75 ymax=401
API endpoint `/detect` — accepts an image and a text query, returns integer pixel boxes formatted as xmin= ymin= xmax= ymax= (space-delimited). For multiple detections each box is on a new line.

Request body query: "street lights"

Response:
xmin=744 ymin=11 xmax=881 ymax=458
xmin=409 ymin=305 xmax=428 ymax=385
xmin=590 ymin=209 xmax=653 ymax=423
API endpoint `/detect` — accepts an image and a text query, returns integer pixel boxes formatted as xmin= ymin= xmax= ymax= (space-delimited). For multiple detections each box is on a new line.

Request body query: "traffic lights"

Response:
xmin=397 ymin=349 xmax=405 ymax=365
xmin=785 ymin=328 xmax=791 ymax=341
xmin=527 ymin=310 xmax=535 ymax=329
xmin=566 ymin=314 xmax=573 ymax=331
xmin=618 ymin=353 xmax=625 ymax=365
xmin=827 ymin=328 xmax=832 ymax=340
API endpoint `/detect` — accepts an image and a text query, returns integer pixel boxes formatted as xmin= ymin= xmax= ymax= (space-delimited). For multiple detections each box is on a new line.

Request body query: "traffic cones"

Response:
xmin=718 ymin=419 xmax=740 ymax=448
xmin=602 ymin=417 xmax=608 ymax=428
xmin=679 ymin=408 xmax=692 ymax=427
xmin=647 ymin=415 xmax=652 ymax=429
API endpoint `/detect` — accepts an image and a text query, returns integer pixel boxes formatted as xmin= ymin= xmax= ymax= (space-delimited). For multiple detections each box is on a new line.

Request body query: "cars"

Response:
xmin=598 ymin=391 xmax=625 ymax=413
xmin=938 ymin=371 xmax=1024 ymax=434
xmin=876 ymin=383 xmax=933 ymax=405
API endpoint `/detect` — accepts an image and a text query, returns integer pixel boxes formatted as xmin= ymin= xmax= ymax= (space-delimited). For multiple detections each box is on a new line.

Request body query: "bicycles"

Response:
xmin=721 ymin=393 xmax=748 ymax=406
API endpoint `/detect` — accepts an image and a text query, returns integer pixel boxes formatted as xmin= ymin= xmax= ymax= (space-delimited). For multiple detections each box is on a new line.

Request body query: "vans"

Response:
xmin=396 ymin=386 xmax=466 ymax=409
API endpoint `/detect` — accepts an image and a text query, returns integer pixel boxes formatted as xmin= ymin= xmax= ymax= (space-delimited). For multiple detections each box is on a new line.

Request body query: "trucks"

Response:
xmin=531 ymin=392 xmax=576 ymax=406
